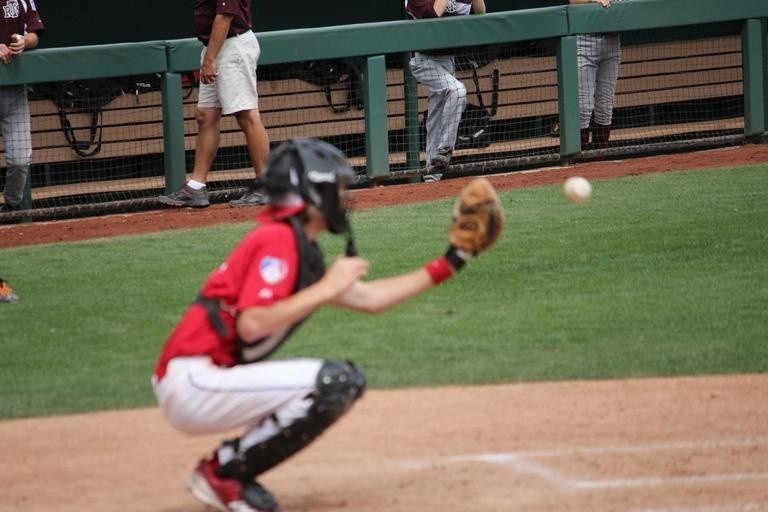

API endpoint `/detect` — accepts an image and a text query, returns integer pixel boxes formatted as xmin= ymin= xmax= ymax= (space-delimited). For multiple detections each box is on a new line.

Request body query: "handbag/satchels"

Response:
xmin=30 ymin=72 xmax=161 ymax=157
xmin=299 ymin=57 xmax=365 ymax=114
xmin=419 ymin=103 xmax=490 ymax=152
xmin=256 ymin=62 xmax=300 ymax=81
xmin=181 ymin=70 xmax=200 ymax=100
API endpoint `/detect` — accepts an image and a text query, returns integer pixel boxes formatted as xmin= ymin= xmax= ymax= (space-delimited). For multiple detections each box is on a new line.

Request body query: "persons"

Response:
xmin=403 ymin=0 xmax=489 ymax=183
xmin=146 ymin=136 xmax=507 ymax=512
xmin=568 ymin=0 xmax=625 ymax=167
xmin=154 ymin=0 xmax=271 ymax=210
xmin=1 ymin=0 xmax=47 ymax=226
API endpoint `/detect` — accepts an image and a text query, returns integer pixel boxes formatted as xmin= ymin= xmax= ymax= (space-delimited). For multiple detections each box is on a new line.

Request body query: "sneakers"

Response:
xmin=183 ymin=449 xmax=277 ymax=512
xmin=228 ymin=176 xmax=271 ymax=207
xmin=157 ymin=181 xmax=211 ymax=207
xmin=431 ymin=145 xmax=453 ymax=168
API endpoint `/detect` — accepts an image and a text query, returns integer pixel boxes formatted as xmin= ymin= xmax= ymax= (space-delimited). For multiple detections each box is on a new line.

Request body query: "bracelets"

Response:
xmin=425 ymin=256 xmax=454 ymax=287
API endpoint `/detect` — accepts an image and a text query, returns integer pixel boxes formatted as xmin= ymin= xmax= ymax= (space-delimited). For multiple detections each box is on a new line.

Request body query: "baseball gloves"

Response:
xmin=446 ymin=176 xmax=505 ymax=256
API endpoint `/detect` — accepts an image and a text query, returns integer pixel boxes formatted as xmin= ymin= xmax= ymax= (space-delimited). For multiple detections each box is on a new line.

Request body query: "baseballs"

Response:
xmin=561 ymin=175 xmax=591 ymax=205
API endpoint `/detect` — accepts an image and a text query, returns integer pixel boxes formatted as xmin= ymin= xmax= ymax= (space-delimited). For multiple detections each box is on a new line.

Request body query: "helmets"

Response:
xmin=257 ymin=135 xmax=357 ymax=233
